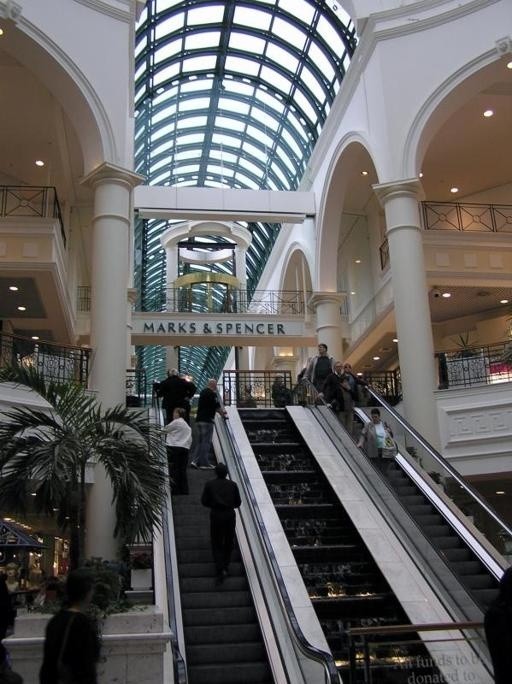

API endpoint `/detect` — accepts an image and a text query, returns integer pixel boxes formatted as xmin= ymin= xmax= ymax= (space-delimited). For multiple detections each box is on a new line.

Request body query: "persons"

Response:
xmin=152 ymin=367 xmax=197 ymax=423
xmin=38 ymin=572 xmax=103 ymax=684
xmin=356 ymin=408 xmax=394 ymax=476
xmin=155 ymin=405 xmax=194 ymax=495
xmin=190 ymin=378 xmax=231 ymax=471
xmin=272 ymin=347 xmax=366 ymax=441
xmin=201 ymin=462 xmax=242 ymax=580
xmin=1 ymin=570 xmax=25 ymax=683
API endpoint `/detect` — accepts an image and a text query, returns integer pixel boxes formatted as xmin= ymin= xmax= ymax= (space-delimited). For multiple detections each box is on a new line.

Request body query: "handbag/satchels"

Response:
xmin=59 ymin=665 xmax=78 ymax=683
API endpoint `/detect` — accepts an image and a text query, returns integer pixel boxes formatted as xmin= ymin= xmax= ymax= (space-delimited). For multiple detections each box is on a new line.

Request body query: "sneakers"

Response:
xmin=190 ymin=461 xmax=217 ymax=470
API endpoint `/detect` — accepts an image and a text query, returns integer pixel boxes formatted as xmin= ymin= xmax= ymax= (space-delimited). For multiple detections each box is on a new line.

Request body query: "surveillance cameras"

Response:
xmin=433 ymin=289 xmax=440 ymax=297
